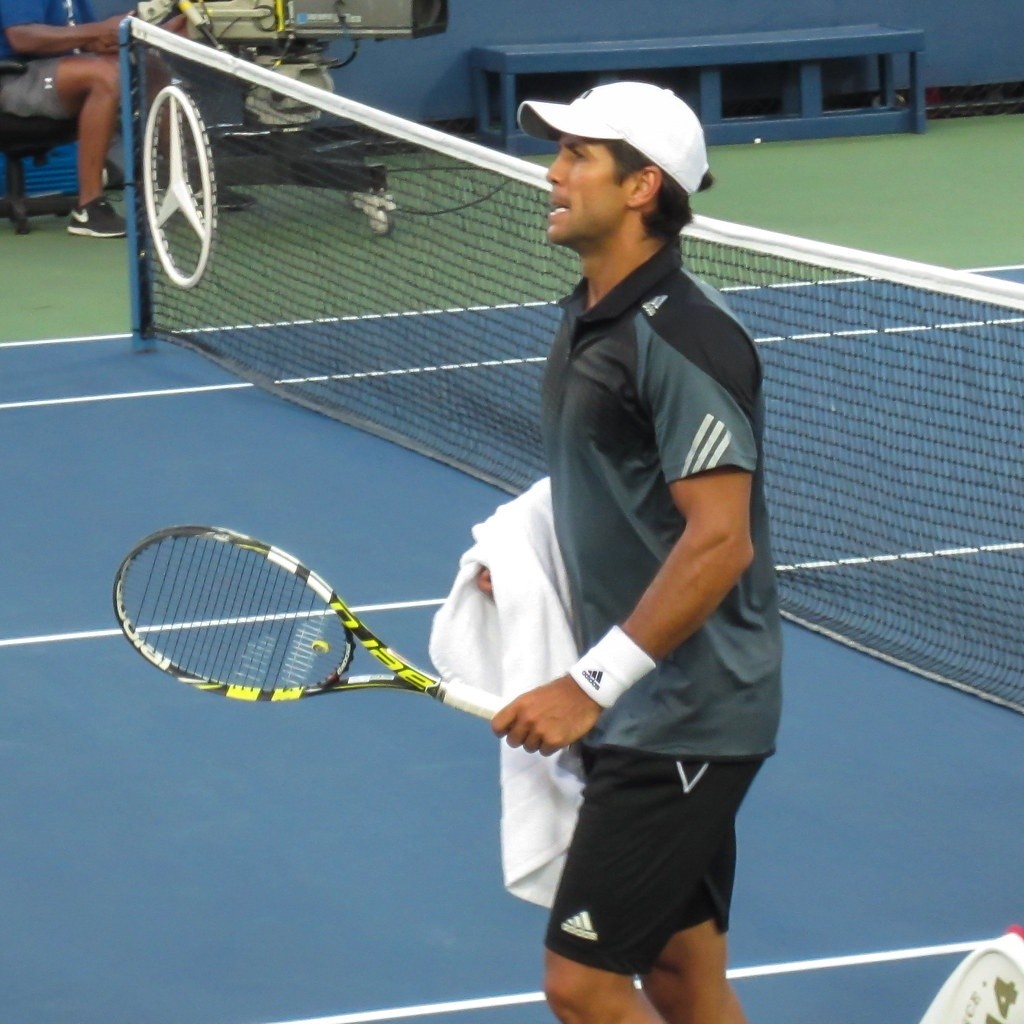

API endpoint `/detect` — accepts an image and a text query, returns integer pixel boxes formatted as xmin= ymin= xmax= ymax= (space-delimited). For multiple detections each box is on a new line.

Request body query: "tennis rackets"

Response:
xmin=114 ymin=527 xmax=518 ymax=727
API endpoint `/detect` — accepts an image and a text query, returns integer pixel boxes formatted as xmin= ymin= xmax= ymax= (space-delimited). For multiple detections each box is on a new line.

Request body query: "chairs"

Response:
xmin=0 ymin=58 xmax=79 ymax=235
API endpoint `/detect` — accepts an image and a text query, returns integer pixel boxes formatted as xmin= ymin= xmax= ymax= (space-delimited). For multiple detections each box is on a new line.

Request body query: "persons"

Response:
xmin=478 ymin=80 xmax=784 ymax=1024
xmin=0 ymin=0 xmax=257 ymax=239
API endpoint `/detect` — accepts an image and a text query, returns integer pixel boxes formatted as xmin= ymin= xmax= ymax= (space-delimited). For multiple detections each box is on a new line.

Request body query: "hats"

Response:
xmin=517 ymin=81 xmax=708 ymax=194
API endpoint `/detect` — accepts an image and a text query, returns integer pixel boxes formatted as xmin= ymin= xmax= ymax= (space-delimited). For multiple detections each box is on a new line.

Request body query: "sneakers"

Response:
xmin=191 ymin=178 xmax=258 ymax=210
xmin=68 ymin=196 xmax=127 ymax=238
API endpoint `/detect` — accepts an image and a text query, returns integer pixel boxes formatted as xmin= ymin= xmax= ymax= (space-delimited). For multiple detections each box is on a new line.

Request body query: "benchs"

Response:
xmin=468 ymin=24 xmax=927 ymax=156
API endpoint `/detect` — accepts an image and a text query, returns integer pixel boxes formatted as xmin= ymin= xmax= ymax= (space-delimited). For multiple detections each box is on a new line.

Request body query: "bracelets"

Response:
xmin=569 ymin=624 xmax=657 ymax=712
